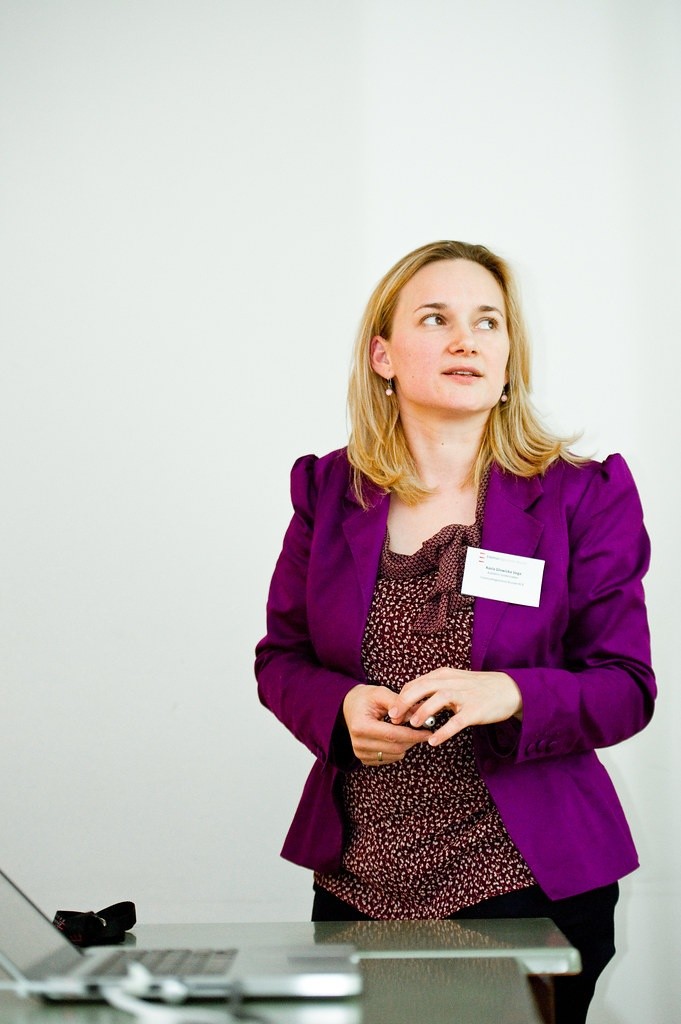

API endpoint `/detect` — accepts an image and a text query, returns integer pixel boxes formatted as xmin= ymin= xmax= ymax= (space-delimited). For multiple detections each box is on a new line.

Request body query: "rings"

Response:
xmin=377 ymin=751 xmax=384 ymax=762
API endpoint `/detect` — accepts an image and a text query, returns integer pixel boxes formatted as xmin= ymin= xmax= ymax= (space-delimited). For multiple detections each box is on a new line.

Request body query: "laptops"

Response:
xmin=2 ymin=870 xmax=364 ymax=1007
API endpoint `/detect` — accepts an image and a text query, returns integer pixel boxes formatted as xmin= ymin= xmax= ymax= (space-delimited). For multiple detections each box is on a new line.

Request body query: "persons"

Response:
xmin=253 ymin=240 xmax=658 ymax=1024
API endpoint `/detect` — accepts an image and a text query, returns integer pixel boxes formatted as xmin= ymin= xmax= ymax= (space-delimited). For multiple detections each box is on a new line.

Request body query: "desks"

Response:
xmin=39 ymin=919 xmax=580 ymax=1024
xmin=0 ymin=960 xmax=548 ymax=1024
